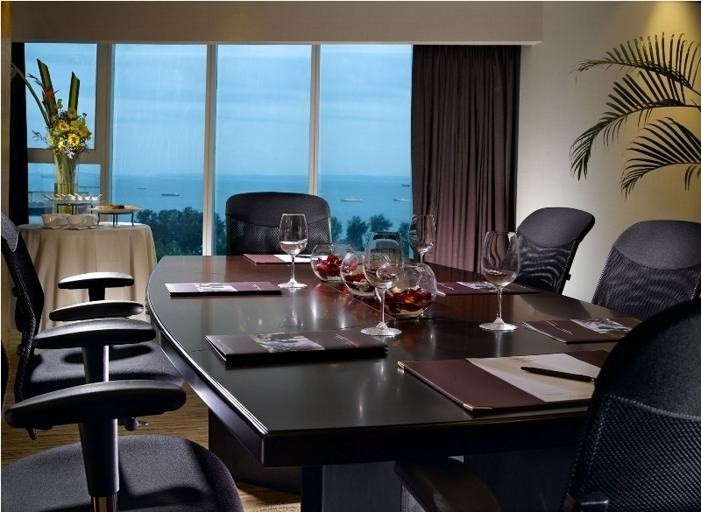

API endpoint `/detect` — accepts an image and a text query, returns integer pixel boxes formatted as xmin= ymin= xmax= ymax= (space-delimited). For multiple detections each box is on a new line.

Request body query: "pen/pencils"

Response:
xmin=520 ymin=366 xmax=596 ymax=384
xmin=294 ymin=255 xmax=310 ymax=258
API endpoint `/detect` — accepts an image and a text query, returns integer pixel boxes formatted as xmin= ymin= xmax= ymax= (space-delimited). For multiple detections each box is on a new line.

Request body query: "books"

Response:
xmin=435 ymin=279 xmax=539 ymax=295
xmin=165 ymin=281 xmax=281 ymax=297
xmin=204 ymin=329 xmax=387 ymax=362
xmin=522 ymin=315 xmax=642 ymax=343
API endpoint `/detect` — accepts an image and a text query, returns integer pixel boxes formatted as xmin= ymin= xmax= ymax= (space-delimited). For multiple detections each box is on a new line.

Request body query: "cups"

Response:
xmin=71 ymin=202 xmax=98 ymax=229
xmin=42 ymin=203 xmax=69 ymax=229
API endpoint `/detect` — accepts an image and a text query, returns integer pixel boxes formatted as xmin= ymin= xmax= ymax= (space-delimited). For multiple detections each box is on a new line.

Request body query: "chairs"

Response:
xmin=1 ymin=317 xmax=243 ymax=512
xmin=225 ymin=191 xmax=332 ymax=256
xmin=501 ymin=207 xmax=595 ymax=295
xmin=393 ymin=299 xmax=701 ymax=511
xmin=591 ymin=219 xmax=701 ymax=319
xmin=1 ymin=210 xmax=185 ymax=441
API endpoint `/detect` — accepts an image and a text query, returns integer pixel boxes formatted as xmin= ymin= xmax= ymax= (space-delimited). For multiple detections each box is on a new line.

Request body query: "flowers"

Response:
xmin=10 ymin=58 xmax=92 ymax=160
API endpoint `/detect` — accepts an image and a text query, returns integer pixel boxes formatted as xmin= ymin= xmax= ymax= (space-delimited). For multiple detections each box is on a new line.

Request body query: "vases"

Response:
xmin=53 ymin=160 xmax=78 ymax=216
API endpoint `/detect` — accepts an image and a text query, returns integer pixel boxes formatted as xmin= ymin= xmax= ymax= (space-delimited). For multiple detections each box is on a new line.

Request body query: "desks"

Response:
xmin=146 ymin=255 xmax=642 ymax=511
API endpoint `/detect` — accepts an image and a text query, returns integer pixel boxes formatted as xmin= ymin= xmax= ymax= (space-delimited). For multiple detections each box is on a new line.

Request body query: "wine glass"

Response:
xmin=362 ymin=238 xmax=403 ymax=335
xmin=409 ymin=214 xmax=434 ymax=268
xmin=280 ymin=214 xmax=309 ymax=289
xmin=479 ymin=231 xmax=520 ymax=333
